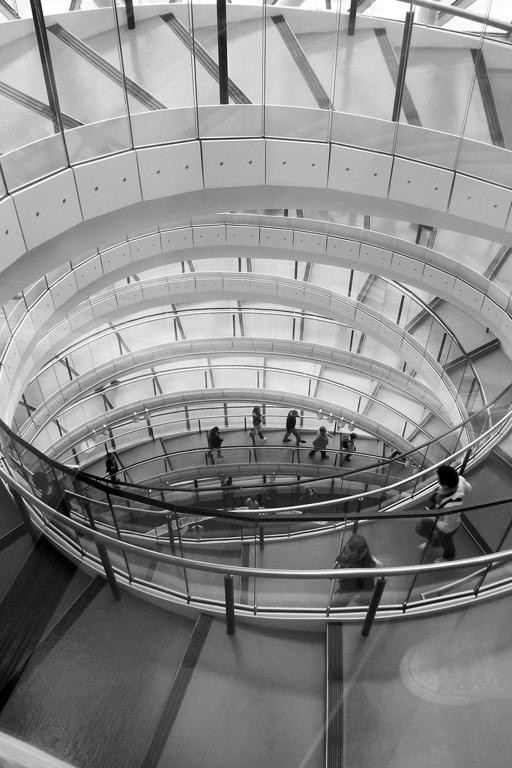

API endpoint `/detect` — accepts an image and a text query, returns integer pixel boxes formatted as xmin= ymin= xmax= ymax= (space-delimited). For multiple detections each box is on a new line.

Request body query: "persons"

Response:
xmin=330 ymin=535 xmax=384 ymax=607
xmin=225 ymin=477 xmax=311 ymax=514
xmin=283 ymin=410 xmax=306 ymax=444
xmin=32 ymin=471 xmax=72 ymax=519
xmin=71 ymin=468 xmax=83 ymax=491
xmin=418 ymin=465 xmax=472 ymax=563
xmin=249 ymin=406 xmax=267 ymax=441
xmin=208 ymin=426 xmax=223 ymax=458
xmin=389 ymin=448 xmax=406 ymax=463
xmin=310 ymin=425 xmax=333 ymax=458
xmin=105 ymin=452 xmax=120 ymax=482
xmin=344 ymin=433 xmax=357 ymax=461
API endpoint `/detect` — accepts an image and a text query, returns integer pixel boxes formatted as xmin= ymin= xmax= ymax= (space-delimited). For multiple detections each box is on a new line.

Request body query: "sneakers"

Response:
xmin=207 ymin=453 xmax=224 ymax=459
xmin=345 ymin=456 xmax=351 ymax=462
xmin=283 ymin=438 xmax=307 ymax=443
xmin=249 ymin=432 xmax=268 ymax=442
xmin=432 ymin=553 xmax=449 ymax=563
xmin=418 ymin=542 xmax=440 ymax=553
xmin=308 ymin=452 xmax=330 ymax=459
